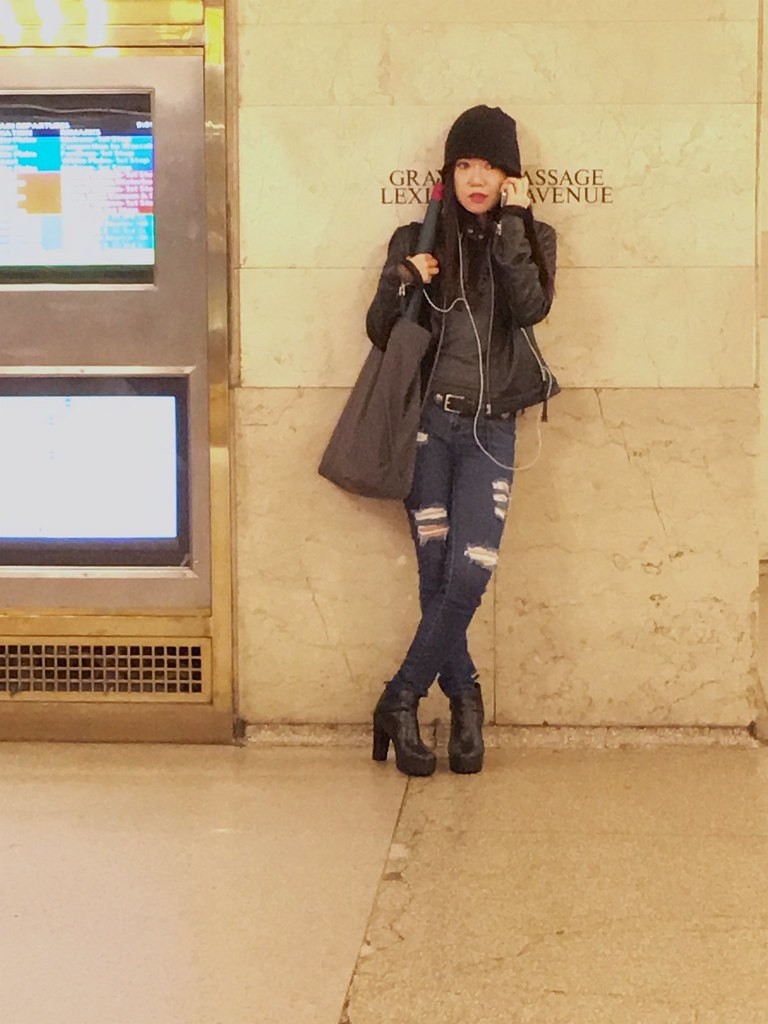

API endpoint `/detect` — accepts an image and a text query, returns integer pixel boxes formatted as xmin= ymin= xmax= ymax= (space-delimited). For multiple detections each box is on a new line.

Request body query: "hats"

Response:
xmin=444 ymin=105 xmax=522 ymax=177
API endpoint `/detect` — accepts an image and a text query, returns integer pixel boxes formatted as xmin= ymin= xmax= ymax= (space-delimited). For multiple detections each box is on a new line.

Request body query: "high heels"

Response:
xmin=447 ymin=682 xmax=485 ymax=773
xmin=371 ymin=679 xmax=436 ymax=776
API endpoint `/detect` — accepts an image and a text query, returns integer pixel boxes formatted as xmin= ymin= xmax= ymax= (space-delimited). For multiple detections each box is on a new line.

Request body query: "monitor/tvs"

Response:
xmin=0 ymin=95 xmax=156 ymax=285
xmin=0 ymin=377 xmax=191 ymax=568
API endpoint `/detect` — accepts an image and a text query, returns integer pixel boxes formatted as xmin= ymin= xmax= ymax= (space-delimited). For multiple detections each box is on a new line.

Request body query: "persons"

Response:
xmin=366 ymin=104 xmax=558 ymax=775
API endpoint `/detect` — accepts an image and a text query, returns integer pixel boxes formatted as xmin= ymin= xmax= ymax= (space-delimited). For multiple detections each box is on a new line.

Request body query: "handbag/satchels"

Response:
xmin=318 ymin=258 xmax=431 ymax=501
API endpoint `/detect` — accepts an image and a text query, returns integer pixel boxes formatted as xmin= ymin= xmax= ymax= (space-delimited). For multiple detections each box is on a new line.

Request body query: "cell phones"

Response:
xmin=500 ymin=193 xmax=508 ymax=208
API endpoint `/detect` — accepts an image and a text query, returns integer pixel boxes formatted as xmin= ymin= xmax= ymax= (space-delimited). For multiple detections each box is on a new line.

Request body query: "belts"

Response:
xmin=430 ymin=391 xmax=516 ymax=422
